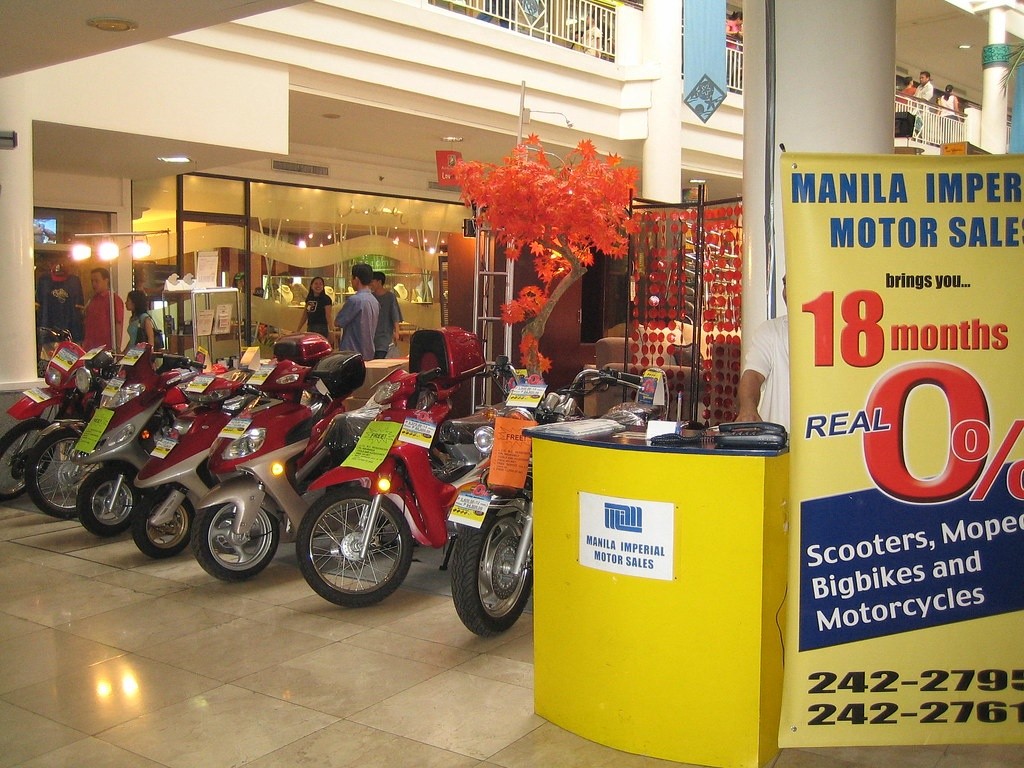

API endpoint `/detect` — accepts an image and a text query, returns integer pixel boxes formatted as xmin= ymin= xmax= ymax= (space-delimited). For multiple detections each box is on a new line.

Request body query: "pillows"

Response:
xmin=633 ymin=323 xmax=686 ymax=366
xmin=669 ymin=341 xmax=704 ymax=366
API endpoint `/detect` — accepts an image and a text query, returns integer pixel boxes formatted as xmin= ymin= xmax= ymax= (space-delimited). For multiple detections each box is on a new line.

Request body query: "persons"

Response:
xmin=335 ymin=263 xmax=404 ymax=359
xmin=896 ymin=71 xmax=934 ymax=104
xmin=726 ymin=10 xmax=743 ymax=50
xmin=296 ymin=277 xmax=334 ymax=339
xmin=939 ymin=84 xmax=960 ymax=121
xmin=123 ymin=290 xmax=164 ymax=355
xmin=569 ymin=12 xmax=601 ymax=58
xmin=476 ymin=0 xmax=509 ymax=28
xmin=736 ymin=274 xmax=792 ymax=436
xmin=81 ymin=267 xmax=124 ymax=353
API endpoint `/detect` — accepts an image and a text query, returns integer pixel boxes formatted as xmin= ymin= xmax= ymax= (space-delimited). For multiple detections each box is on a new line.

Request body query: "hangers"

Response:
xmin=50 ymin=264 xmax=69 ymax=274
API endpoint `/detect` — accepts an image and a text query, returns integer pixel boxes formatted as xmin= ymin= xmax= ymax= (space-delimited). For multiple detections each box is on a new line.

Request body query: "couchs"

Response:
xmin=594 ymin=336 xmax=706 ymax=423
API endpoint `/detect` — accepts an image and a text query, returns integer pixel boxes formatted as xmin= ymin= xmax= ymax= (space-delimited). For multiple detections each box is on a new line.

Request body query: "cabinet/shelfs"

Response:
xmin=162 ymin=287 xmax=242 ymax=374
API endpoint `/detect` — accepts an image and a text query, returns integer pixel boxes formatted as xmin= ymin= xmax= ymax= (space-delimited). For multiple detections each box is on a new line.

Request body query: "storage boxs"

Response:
xmin=894 ymin=111 xmax=915 ymax=137
xmin=353 ymin=359 xmax=410 ymax=397
xmin=37 ymin=341 xmax=60 ymax=362
xmin=344 ymin=398 xmax=368 ymax=410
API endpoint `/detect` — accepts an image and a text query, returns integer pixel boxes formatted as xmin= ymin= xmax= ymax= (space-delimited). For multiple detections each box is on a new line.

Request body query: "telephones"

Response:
xmin=714 ymin=422 xmax=788 ymax=450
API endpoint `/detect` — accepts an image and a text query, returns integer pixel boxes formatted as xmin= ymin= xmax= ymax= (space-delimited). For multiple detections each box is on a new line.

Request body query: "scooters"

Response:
xmin=77 ymin=334 xmax=522 ymax=611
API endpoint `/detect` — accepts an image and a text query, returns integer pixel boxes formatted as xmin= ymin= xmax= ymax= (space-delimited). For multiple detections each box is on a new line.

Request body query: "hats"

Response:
xmin=51 ymin=259 xmax=69 ymax=282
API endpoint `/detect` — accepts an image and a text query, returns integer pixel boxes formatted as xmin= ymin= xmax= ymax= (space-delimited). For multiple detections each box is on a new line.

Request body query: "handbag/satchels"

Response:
xmin=134 ymin=312 xmax=164 ymax=348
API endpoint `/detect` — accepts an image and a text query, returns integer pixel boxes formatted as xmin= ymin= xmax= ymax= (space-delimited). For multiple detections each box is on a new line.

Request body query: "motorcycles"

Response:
xmin=0 ymin=329 xmax=123 ymax=521
xmin=451 ymin=353 xmax=671 ymax=639
